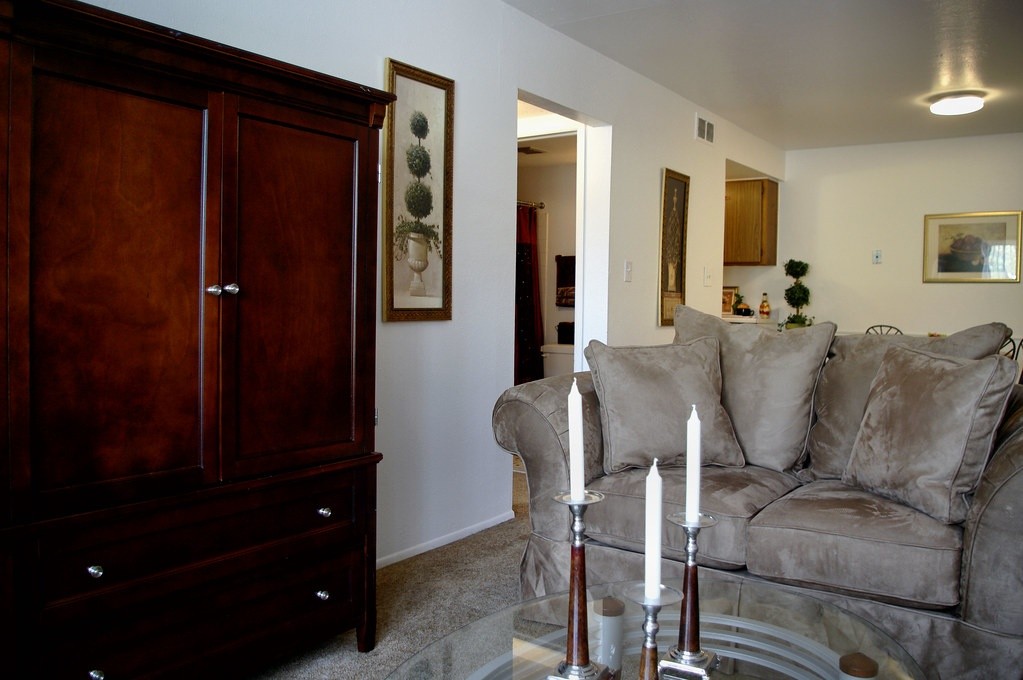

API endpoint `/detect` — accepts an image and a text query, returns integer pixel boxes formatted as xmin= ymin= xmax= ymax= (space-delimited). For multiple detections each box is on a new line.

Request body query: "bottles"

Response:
xmin=759 ymin=293 xmax=770 ymax=319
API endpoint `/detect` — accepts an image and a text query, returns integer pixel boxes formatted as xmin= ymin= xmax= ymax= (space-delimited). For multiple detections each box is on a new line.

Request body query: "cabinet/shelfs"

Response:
xmin=0 ymin=0 xmax=397 ymax=679
xmin=725 ymin=177 xmax=777 ymax=266
xmin=722 ymin=286 xmax=739 ymax=315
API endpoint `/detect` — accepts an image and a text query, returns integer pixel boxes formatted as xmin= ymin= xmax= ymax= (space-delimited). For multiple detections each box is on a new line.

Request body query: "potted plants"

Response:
xmin=778 ymin=259 xmax=813 ymax=331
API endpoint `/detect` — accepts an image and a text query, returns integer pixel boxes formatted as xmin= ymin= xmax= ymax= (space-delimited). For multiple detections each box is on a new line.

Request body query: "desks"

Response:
xmin=388 ymin=577 xmax=931 ymax=680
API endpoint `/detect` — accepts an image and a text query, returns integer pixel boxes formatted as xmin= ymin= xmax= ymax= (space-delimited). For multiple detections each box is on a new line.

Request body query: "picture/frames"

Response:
xmin=380 ymin=56 xmax=456 ymax=322
xmin=923 ymin=210 xmax=1022 ymax=283
xmin=657 ymin=167 xmax=689 ymax=326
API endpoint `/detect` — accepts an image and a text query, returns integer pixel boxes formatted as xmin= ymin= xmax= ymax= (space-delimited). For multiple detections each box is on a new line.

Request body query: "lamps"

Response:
xmin=929 ymin=94 xmax=986 ymax=115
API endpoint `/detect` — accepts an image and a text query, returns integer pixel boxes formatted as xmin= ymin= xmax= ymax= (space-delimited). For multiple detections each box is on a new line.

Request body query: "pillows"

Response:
xmin=673 ymin=303 xmax=838 ymax=476
xmin=582 ymin=340 xmax=748 ymax=474
xmin=839 ymin=344 xmax=1022 ymax=523
xmin=795 ymin=322 xmax=1014 ymax=483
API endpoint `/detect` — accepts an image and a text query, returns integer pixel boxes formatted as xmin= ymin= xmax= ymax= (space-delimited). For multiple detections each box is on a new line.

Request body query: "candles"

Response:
xmin=684 ymin=404 xmax=702 ymax=523
xmin=567 ymin=378 xmax=584 ymax=501
xmin=644 ymin=458 xmax=661 ymax=601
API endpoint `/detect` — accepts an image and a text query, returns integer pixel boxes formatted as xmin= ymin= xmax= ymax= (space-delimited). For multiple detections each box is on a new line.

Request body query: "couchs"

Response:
xmin=490 ymin=382 xmax=1022 ymax=680
